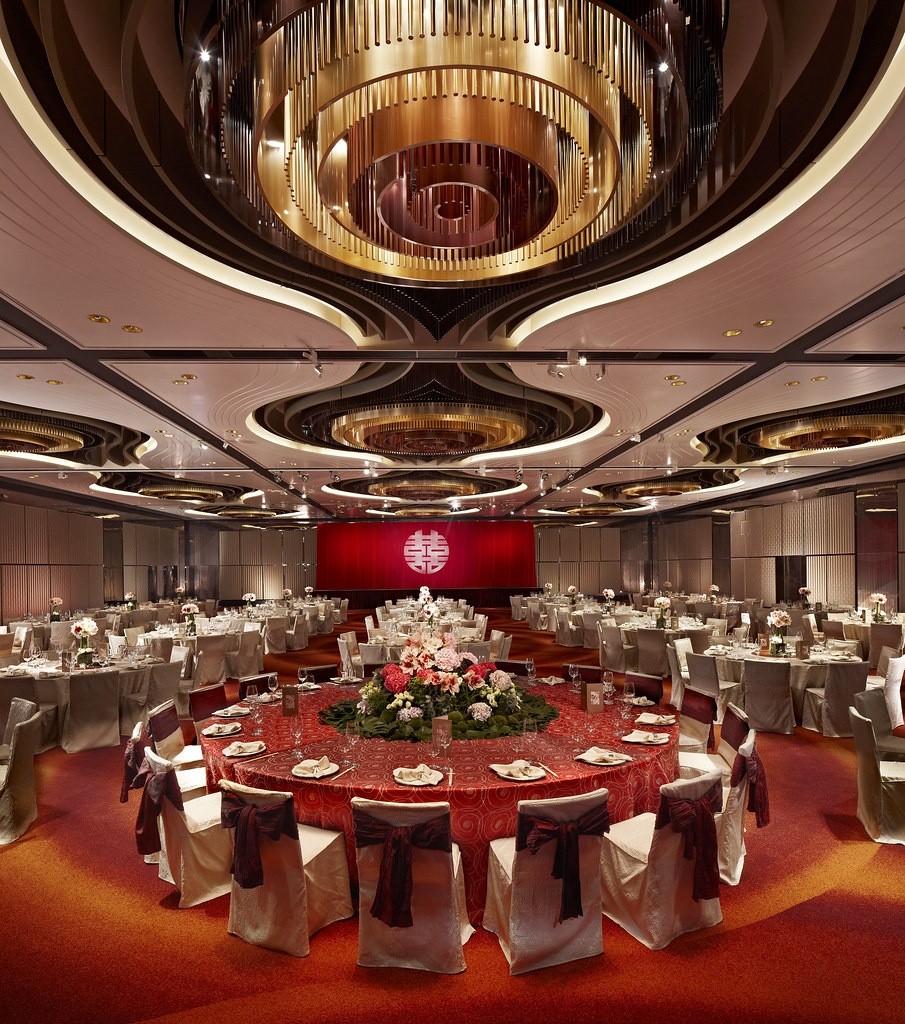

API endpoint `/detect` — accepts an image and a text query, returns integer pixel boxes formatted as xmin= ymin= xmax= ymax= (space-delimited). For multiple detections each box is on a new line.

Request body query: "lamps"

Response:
xmin=0 ymin=0 xmax=905 ymax=531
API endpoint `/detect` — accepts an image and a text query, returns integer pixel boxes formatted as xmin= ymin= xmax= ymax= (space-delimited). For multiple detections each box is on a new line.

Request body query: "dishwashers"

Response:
xmin=479 ymin=656 xmax=486 ymax=663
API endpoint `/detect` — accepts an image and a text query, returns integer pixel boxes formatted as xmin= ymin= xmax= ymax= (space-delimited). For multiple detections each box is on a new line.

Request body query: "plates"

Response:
xmin=394 ymin=770 xmax=443 ymax=785
xmin=588 ymin=759 xmax=625 ymax=765
xmin=222 ymin=746 xmax=266 ymax=756
xmin=633 ymin=698 xmax=675 ymax=725
xmin=497 ymin=766 xmax=545 ymax=780
xmin=218 ymin=715 xmax=244 ymax=717
xmin=210 ymin=727 xmax=241 ymax=735
xmin=291 ymin=761 xmax=339 ymax=777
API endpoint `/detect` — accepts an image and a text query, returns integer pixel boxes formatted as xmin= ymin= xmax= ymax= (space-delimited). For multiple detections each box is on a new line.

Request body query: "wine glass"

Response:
xmin=618 ymin=682 xmax=635 ymax=735
xmin=525 ymin=657 xmax=537 ymax=687
xmin=437 ymin=728 xmax=452 ymax=771
xmin=297 ymin=667 xmax=314 ymax=694
xmin=727 ymin=631 xmax=835 ymax=662
xmin=346 ymin=721 xmax=361 ymax=768
xmin=245 ymin=684 xmax=263 ymax=736
xmin=425 ymin=733 xmax=440 ymax=770
xmin=603 ymin=671 xmax=615 ymax=705
xmin=568 ymin=664 xmax=582 ymax=694
xmin=289 ymin=714 xmax=303 ymax=760
xmin=339 ymin=661 xmax=356 ymax=694
xmin=572 ymin=711 xmax=597 ymax=752
xmin=22 ymin=639 xmax=139 ymax=671
xmin=267 ymin=674 xmax=283 ymax=707
xmin=337 ymin=732 xmax=351 ymax=766
xmin=512 ymin=718 xmax=548 ymax=764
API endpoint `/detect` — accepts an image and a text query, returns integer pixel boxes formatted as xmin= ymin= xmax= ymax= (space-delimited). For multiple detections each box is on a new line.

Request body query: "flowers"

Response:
xmin=51 ymin=580 xmax=888 ymax=739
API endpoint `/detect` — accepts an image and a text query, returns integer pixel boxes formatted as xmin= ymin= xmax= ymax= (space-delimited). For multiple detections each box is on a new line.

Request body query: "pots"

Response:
xmin=642 ymin=738 xmax=669 ymax=743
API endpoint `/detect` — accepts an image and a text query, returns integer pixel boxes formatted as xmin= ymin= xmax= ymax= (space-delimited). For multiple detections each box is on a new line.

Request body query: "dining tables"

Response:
xmin=0 ymin=596 xmax=902 ymax=924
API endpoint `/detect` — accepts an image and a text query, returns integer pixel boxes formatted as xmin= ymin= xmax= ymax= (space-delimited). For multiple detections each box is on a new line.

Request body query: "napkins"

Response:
xmin=634 ymin=712 xmax=678 ymax=726
xmin=392 ymin=763 xmax=441 ymax=785
xmin=212 ymin=706 xmax=251 ymax=717
xmin=201 ymin=721 xmax=242 ymax=735
xmin=487 ymin=763 xmax=547 ymax=779
xmin=574 ymin=746 xmax=634 ymax=765
xmin=622 ymin=729 xmax=671 ymax=744
xmin=629 ymin=695 xmax=657 ymax=708
xmin=296 ymin=755 xmax=332 ymax=780
xmin=225 ymin=741 xmax=266 ymax=757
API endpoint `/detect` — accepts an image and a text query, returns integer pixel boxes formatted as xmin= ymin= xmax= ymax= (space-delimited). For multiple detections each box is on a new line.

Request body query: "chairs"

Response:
xmin=1 ymin=594 xmax=904 ymax=976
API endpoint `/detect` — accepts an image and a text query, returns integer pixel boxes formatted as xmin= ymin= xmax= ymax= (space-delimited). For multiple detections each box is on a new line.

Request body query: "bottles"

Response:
xmin=657 ymin=609 xmax=666 ymax=629
